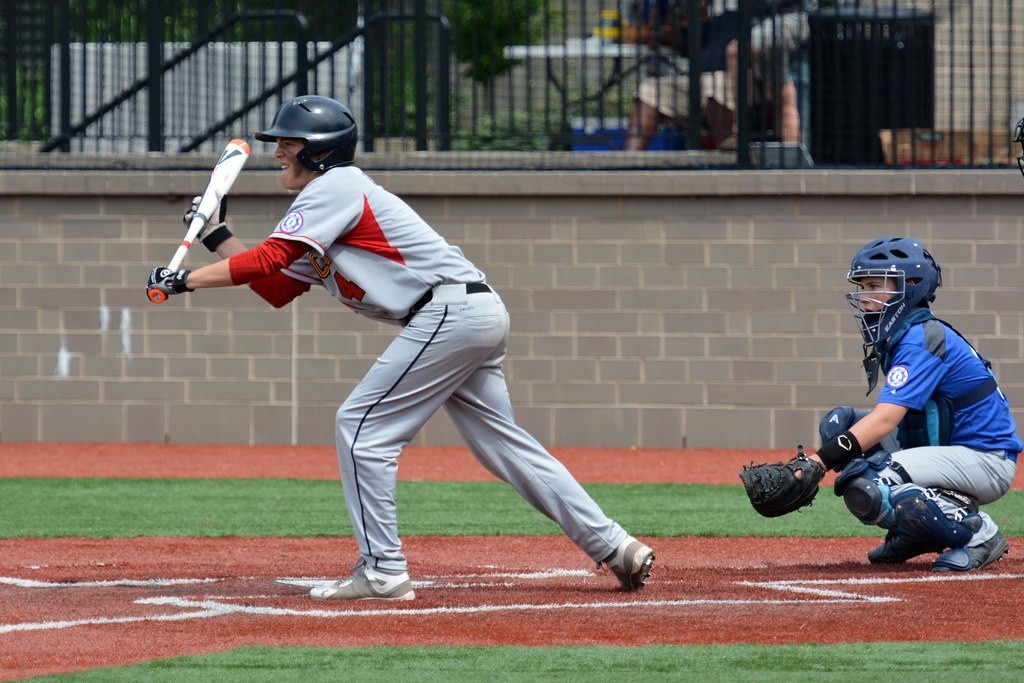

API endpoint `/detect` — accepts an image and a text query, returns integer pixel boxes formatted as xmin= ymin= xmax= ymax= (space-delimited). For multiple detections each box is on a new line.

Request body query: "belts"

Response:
xmin=409 ymin=283 xmax=492 ymax=316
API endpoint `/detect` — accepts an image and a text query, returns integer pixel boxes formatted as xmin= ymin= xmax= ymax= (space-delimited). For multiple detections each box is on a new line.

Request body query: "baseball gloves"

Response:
xmin=737 ymin=444 xmax=826 ymax=519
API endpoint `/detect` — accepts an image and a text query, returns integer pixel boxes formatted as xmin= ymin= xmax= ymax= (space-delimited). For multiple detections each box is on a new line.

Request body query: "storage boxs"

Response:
xmin=571 ymin=118 xmax=683 ymax=152
xmin=880 ymin=127 xmax=1023 ymax=167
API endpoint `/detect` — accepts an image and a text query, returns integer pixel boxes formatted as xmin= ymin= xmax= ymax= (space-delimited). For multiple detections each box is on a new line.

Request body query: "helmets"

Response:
xmin=253 ymin=95 xmax=358 ymax=174
xmin=842 ymin=235 xmax=942 ymax=347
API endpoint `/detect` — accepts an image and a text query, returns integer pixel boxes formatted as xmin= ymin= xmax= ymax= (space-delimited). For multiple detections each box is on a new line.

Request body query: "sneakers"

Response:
xmin=932 ymin=510 xmax=1011 ymax=573
xmin=867 ymin=530 xmax=948 ymax=565
xmin=308 ymin=556 xmax=416 ymax=601
xmin=602 ymin=535 xmax=656 ymax=591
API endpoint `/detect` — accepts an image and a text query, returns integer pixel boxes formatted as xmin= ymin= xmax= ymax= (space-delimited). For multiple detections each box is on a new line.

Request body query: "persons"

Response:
xmin=144 ymin=94 xmax=656 ymax=602
xmin=627 ymin=1 xmax=810 ymax=151
xmin=740 ymin=237 xmax=1024 ymax=573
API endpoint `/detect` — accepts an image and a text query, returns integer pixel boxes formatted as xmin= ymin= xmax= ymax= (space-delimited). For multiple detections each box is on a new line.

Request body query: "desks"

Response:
xmin=505 ymin=44 xmax=676 ymax=150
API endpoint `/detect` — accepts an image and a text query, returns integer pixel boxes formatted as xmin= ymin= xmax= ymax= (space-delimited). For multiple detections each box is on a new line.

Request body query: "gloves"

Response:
xmin=182 ymin=193 xmax=233 ymax=252
xmin=144 ymin=265 xmax=194 ymax=302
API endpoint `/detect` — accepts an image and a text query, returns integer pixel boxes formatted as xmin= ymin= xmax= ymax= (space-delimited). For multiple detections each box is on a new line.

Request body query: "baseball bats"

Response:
xmin=146 ymin=138 xmax=252 ymax=304
